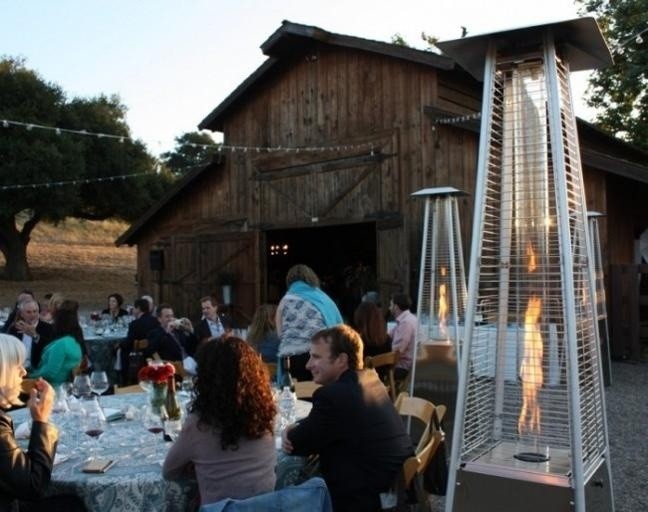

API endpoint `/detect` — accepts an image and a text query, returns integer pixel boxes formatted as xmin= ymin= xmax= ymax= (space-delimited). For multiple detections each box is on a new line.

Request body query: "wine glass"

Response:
xmin=142 ymin=407 xmax=165 ymax=466
xmin=60 ymin=383 xmax=75 ymax=418
xmin=79 ymin=393 xmax=109 ymax=464
xmin=73 ymin=375 xmax=92 ymax=414
xmin=88 ymin=369 xmax=109 ymax=408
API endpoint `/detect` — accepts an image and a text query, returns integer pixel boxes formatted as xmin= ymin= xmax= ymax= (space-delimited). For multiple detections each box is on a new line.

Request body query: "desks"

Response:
xmin=384 ymin=313 xmax=560 ymax=386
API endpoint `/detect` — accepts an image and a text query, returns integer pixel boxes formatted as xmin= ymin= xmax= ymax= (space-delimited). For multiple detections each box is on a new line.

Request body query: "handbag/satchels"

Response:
xmin=422 ymin=408 xmax=448 ymax=496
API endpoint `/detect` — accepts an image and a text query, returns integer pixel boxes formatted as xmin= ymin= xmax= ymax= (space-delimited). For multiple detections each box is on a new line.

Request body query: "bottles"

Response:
xmin=279 ymin=386 xmax=296 ymax=426
xmin=280 ymin=355 xmax=296 ymax=405
xmin=163 ymin=374 xmax=181 ymax=442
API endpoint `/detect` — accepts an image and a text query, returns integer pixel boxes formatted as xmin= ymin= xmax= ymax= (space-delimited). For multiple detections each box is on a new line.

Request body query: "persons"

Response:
xmin=274 ymin=263 xmax=345 ymax=405
xmin=3 ymin=292 xmax=419 ymax=411
xmin=1 ymin=333 xmax=88 ymax=511
xmin=282 ymin=323 xmax=425 ymax=512
xmin=163 ymin=335 xmax=278 ymax=512
